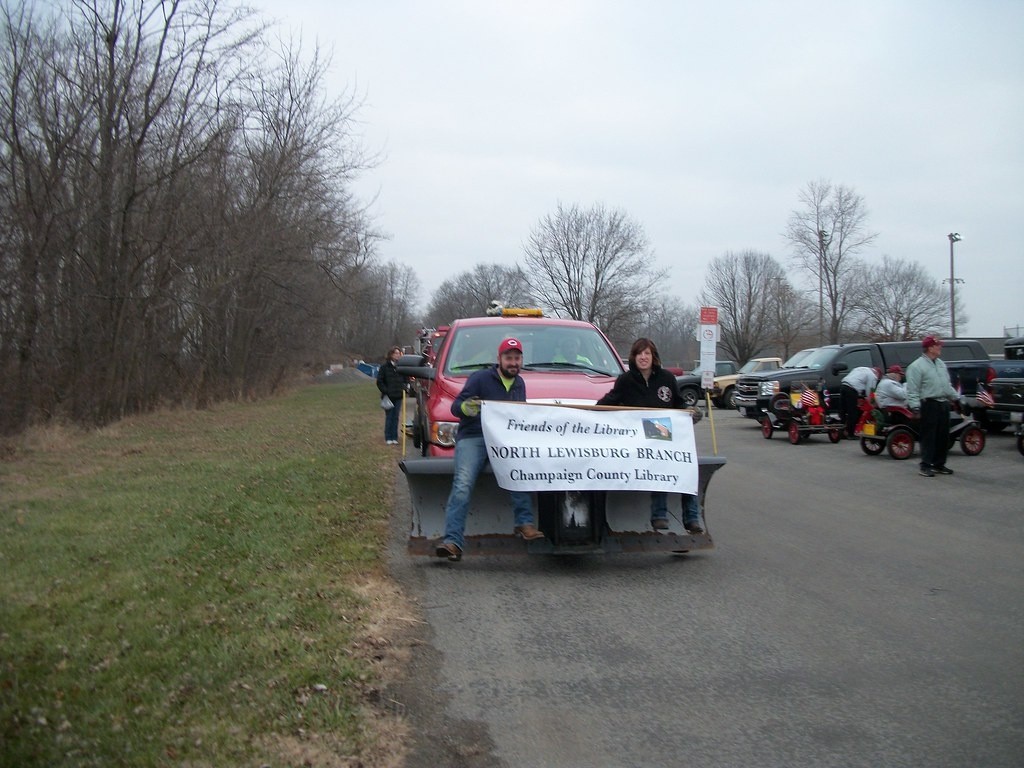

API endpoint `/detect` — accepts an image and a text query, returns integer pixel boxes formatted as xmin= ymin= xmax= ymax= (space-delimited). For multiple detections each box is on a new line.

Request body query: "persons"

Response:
xmin=376 ymin=346 xmax=410 ymax=445
xmin=838 ymin=367 xmax=882 ymax=440
xmin=550 ymin=335 xmax=593 ymax=367
xmin=596 ymin=338 xmax=704 ymax=533
xmin=906 ymin=337 xmax=961 ymax=477
xmin=436 ymin=338 xmax=545 ymax=559
xmin=874 ymin=366 xmax=911 ymax=412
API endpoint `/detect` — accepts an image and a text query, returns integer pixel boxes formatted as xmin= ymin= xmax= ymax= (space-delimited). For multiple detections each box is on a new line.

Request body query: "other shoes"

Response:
xmin=651 ymin=519 xmax=669 ymax=529
xmin=386 ymin=440 xmax=399 ymax=445
xmin=918 ymin=462 xmax=935 ymax=477
xmin=685 ymin=522 xmax=703 ymax=533
xmin=846 ymin=434 xmax=860 ymax=440
xmin=931 ymin=464 xmax=953 ymax=474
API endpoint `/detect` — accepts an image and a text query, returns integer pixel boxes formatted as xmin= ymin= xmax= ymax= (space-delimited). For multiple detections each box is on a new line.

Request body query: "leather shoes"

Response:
xmin=435 ymin=542 xmax=462 ymax=563
xmin=513 ymin=524 xmax=545 ymax=541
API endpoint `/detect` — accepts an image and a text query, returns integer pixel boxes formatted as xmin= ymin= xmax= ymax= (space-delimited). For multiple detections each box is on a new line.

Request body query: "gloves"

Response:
xmin=461 ymin=396 xmax=481 ymax=418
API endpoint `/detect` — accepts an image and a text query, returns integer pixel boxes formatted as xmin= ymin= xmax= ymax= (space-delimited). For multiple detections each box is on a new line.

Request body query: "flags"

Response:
xmin=801 ymin=382 xmax=818 ymax=407
xmin=976 ymin=382 xmax=995 ymax=407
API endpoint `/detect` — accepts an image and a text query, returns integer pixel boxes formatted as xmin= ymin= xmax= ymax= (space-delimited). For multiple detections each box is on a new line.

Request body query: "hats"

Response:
xmin=886 ymin=365 xmax=904 ymax=375
xmin=922 ymin=335 xmax=944 ymax=347
xmin=498 ymin=338 xmax=523 ymax=355
xmin=873 ymin=367 xmax=882 ymax=379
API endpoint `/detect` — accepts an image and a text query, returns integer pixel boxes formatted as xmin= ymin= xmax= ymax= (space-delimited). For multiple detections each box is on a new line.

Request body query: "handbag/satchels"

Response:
xmin=380 ymin=394 xmax=395 ymax=410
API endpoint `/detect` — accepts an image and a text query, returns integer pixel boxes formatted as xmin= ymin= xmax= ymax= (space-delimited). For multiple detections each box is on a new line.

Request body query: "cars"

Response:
xmin=621 ymin=358 xmax=683 ymax=377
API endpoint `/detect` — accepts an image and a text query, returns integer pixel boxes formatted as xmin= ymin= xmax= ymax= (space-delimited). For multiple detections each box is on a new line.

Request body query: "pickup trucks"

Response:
xmin=676 ymin=360 xmax=740 ymax=406
xmin=706 ymin=357 xmax=783 ymax=409
xmin=946 ymin=361 xmax=1024 ymax=430
xmin=731 ymin=341 xmax=991 ymax=426
xmin=395 ymin=306 xmax=725 ymax=558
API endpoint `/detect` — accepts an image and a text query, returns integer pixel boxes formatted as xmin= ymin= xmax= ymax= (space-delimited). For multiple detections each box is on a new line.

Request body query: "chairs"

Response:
xmin=770 ymin=391 xmax=794 ymax=417
xmin=883 ymin=406 xmax=913 ymax=424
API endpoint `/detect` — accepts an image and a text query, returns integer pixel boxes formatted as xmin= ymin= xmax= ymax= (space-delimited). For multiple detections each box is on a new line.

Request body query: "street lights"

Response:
xmin=943 ymin=232 xmax=964 ymax=339
